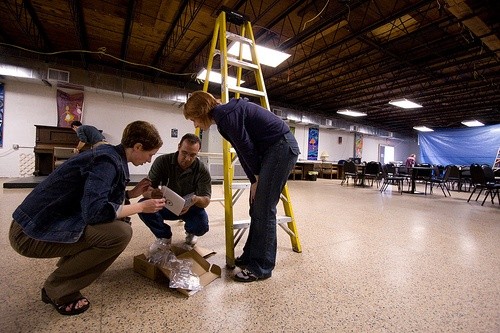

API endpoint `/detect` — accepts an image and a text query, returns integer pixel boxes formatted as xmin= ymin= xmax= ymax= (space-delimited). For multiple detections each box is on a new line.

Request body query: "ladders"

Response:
xmin=484 ymin=147 xmax=500 ymax=194
xmin=178 ymin=5 xmax=302 ymax=269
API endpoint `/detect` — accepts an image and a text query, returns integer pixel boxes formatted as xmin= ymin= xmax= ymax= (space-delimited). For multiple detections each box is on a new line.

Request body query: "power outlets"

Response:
xmin=12 ymin=144 xmax=19 ymax=150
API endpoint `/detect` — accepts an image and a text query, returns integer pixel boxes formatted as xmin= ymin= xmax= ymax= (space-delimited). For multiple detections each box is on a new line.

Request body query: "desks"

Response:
xmin=296 ymin=161 xmax=318 ymax=179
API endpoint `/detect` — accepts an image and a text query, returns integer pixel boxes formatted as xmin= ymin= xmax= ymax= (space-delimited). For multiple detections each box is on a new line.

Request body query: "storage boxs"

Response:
xmin=132 ymin=244 xmax=222 ymax=299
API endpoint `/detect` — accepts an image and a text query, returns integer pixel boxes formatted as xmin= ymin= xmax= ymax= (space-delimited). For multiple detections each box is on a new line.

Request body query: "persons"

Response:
xmin=10 ymin=121 xmax=166 ymax=316
xmin=71 ymin=120 xmax=109 ymax=154
xmin=183 ymin=91 xmax=301 ymax=283
xmin=406 ymin=154 xmax=415 ymax=182
xmin=137 ymin=133 xmax=212 ymax=251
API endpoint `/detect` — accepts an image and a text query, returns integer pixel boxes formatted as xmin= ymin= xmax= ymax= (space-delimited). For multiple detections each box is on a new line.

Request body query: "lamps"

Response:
xmin=227 ymin=28 xmax=292 ymax=69
xmin=461 ymin=113 xmax=485 ymax=127
xmin=195 ymin=59 xmax=246 ymax=86
xmin=337 ymin=106 xmax=368 ymax=117
xmin=412 ymin=121 xmax=434 ymax=132
xmin=321 ymin=152 xmax=329 ymax=162
xmin=388 ymin=97 xmax=423 ymax=109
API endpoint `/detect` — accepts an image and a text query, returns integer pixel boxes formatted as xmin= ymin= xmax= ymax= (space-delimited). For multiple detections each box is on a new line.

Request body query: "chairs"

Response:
xmin=52 ymin=147 xmax=79 ymax=172
xmin=314 ymin=160 xmax=500 ymax=207
xmin=289 ymin=163 xmax=304 ymax=181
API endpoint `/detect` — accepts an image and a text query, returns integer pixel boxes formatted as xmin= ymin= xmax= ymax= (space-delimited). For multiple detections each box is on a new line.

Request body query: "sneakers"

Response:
xmin=234 ymin=256 xmax=245 ymax=268
xmin=150 ymin=236 xmax=172 ymax=251
xmin=235 ymin=268 xmax=261 ymax=282
xmin=186 ymin=231 xmax=197 ymax=244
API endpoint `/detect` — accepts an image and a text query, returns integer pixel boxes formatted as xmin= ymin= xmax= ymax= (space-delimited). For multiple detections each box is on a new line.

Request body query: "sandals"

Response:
xmin=41 ymin=287 xmax=90 ymax=316
